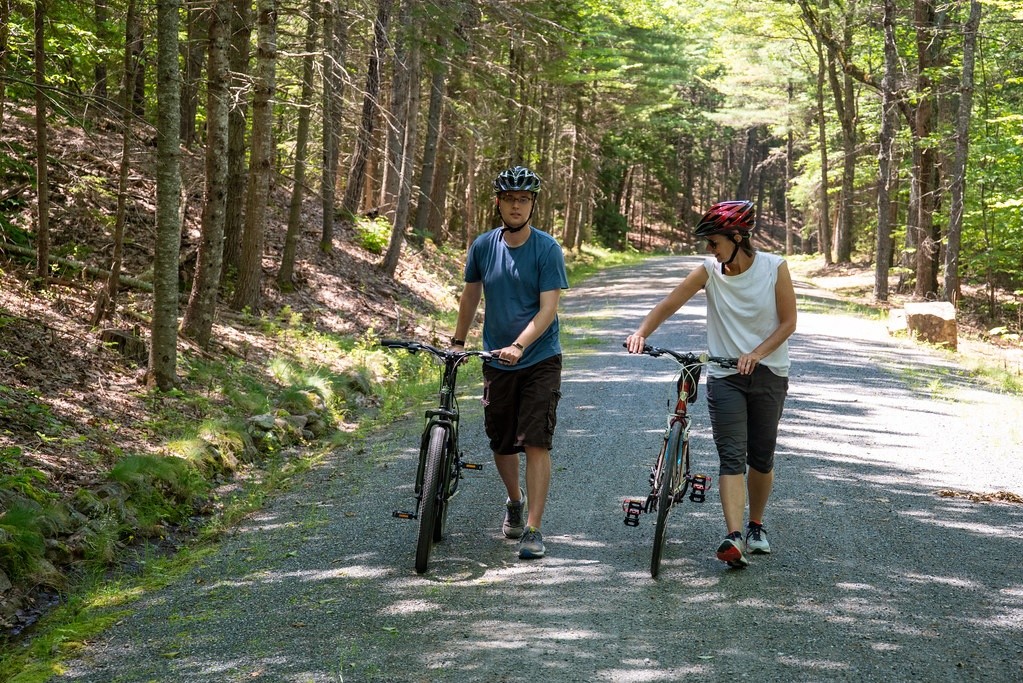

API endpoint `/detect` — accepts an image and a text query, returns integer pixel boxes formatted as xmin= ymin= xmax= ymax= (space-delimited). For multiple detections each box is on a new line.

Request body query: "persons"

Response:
xmin=626 ymin=199 xmax=797 ymax=571
xmin=449 ymin=165 xmax=569 ymax=559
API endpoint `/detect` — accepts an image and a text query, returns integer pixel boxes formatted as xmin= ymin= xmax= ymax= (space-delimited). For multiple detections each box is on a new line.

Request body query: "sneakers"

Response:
xmin=716 ymin=535 xmax=748 ymax=568
xmin=517 ymin=525 xmax=546 ymax=559
xmin=745 ymin=524 xmax=771 ymax=555
xmin=502 ymin=488 xmax=527 ymax=538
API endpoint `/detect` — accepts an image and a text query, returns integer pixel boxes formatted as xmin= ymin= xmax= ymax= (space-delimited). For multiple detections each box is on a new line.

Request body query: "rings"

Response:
xmin=746 ymin=360 xmax=751 ymax=363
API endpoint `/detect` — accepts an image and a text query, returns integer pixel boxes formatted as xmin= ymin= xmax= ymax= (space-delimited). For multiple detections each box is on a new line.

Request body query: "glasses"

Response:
xmin=498 ymin=195 xmax=532 ymax=203
xmin=706 ymin=236 xmax=733 ymax=249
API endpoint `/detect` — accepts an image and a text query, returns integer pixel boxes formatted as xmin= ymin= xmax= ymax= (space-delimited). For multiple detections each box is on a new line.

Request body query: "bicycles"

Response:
xmin=382 ymin=339 xmax=522 ymax=572
xmin=622 ymin=341 xmax=757 ymax=577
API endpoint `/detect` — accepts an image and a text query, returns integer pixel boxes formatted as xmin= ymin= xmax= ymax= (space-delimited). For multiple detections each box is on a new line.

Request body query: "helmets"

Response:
xmin=691 ymin=200 xmax=755 ymax=238
xmin=493 ymin=165 xmax=540 ymax=194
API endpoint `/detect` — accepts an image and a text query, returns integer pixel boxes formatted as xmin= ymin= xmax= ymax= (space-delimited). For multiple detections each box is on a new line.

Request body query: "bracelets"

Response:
xmin=512 ymin=341 xmax=525 ymax=350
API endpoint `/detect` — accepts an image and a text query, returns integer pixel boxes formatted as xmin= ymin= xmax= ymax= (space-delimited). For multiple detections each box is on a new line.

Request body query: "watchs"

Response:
xmin=450 ymin=337 xmax=465 ymax=346
